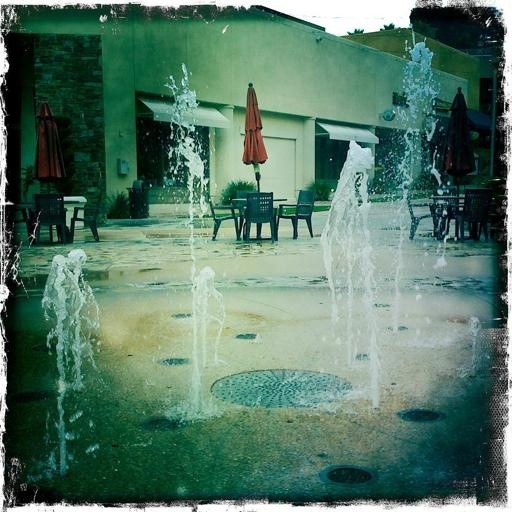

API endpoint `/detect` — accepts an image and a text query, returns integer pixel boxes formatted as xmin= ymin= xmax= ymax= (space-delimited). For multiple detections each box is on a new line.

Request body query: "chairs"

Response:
xmin=9 ymin=192 xmax=104 ymax=243
xmin=405 ymin=192 xmax=488 ymax=244
xmin=205 ymin=190 xmax=318 ymax=243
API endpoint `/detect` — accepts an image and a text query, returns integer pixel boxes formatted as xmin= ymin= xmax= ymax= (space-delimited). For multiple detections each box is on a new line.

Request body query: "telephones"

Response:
xmin=118 ymin=158 xmax=130 ymax=175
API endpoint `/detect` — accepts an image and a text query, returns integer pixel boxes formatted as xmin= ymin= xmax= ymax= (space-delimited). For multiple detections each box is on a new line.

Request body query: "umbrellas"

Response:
xmin=242 ymin=83 xmax=267 ymax=239
xmin=447 ymin=87 xmax=476 ymax=240
xmin=35 ymin=102 xmax=66 ymax=242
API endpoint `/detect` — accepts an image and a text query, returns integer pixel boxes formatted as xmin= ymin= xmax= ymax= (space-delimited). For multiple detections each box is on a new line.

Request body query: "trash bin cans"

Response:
xmin=126 ymin=187 xmax=150 ymax=218
